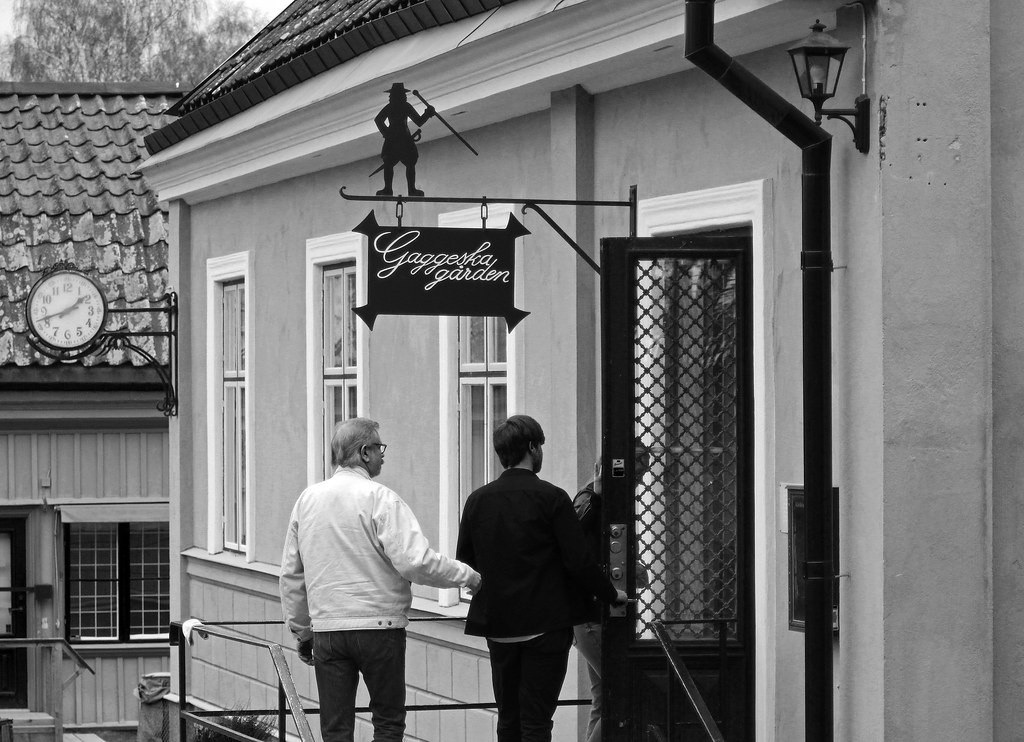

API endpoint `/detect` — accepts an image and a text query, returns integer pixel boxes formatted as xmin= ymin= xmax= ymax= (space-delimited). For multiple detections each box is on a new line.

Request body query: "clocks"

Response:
xmin=23 ymin=260 xmax=110 ymax=352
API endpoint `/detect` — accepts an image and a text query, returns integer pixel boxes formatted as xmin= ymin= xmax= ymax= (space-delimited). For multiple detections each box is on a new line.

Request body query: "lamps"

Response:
xmin=786 ymin=19 xmax=870 ymax=156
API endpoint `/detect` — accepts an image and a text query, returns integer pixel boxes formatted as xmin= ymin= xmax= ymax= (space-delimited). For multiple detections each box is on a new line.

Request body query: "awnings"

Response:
xmin=54 ymin=503 xmax=169 ymax=523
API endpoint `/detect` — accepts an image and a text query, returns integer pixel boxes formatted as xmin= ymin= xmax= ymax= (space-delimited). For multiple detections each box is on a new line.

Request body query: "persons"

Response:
xmin=278 ymin=418 xmax=482 ymax=742
xmin=572 ymin=442 xmax=651 ymax=742
xmin=455 ymin=414 xmax=588 ymax=742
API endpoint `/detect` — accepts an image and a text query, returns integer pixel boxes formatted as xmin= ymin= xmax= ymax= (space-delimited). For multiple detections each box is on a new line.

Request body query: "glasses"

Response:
xmin=358 ymin=443 xmax=387 ymax=454
